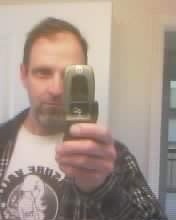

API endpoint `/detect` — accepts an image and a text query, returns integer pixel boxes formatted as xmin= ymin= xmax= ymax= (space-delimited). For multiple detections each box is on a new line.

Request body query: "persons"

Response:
xmin=0 ymin=17 xmax=168 ymax=220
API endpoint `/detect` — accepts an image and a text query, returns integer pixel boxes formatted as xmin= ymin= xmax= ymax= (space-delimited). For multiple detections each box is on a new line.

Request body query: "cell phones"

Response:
xmin=59 ymin=65 xmax=98 ymax=172
